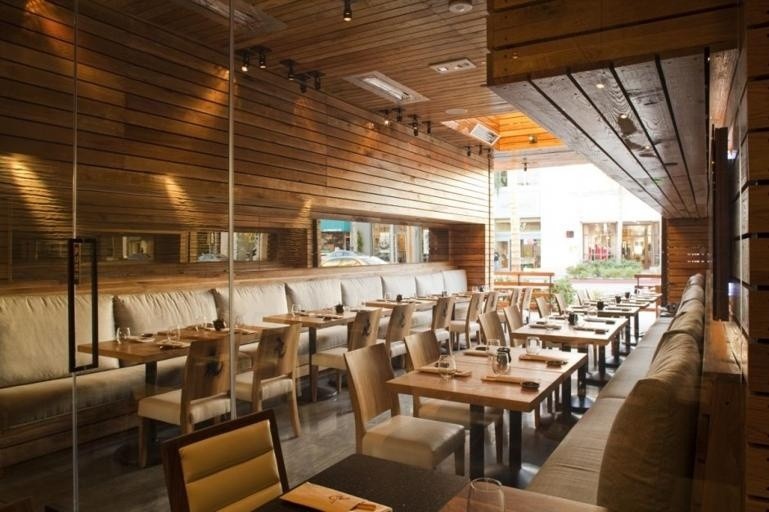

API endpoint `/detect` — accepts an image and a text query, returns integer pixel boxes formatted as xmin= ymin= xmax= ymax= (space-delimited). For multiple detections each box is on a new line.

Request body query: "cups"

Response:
xmin=235 ymin=314 xmax=248 ymax=329
xmin=525 ymin=289 xmax=642 ymax=356
xmin=437 ymin=354 xmax=457 ymax=381
xmin=190 ymin=317 xmax=208 ymax=336
xmin=489 ymin=352 xmax=510 ymax=377
xmin=116 ymin=327 xmax=130 ymax=344
xmin=383 ymin=284 xmax=489 ymax=305
xmin=486 ymin=339 xmax=500 ymax=356
xmin=467 ymin=477 xmax=505 ymax=512
xmin=166 ymin=325 xmax=181 ymax=342
xmin=291 ymin=303 xmax=302 ymax=317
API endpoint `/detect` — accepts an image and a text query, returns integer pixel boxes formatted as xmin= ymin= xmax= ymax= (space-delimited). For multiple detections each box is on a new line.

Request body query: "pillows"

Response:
xmin=596 ymin=273 xmax=705 ymax=512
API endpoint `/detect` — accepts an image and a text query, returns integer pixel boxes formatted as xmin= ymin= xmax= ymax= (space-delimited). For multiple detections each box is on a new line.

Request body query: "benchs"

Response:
xmin=0 ymin=269 xmax=488 ymax=467
xmin=526 ymin=270 xmax=675 ymax=510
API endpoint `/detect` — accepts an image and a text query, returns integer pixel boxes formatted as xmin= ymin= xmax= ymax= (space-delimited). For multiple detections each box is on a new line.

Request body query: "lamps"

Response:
xmin=343 ymin=0 xmax=352 ymax=21
xmin=520 ymin=158 xmax=530 ymax=171
xmin=528 ymin=135 xmax=538 ymax=144
xmin=234 ymin=45 xmax=326 ymax=94
xmin=378 ymin=107 xmax=494 ymax=159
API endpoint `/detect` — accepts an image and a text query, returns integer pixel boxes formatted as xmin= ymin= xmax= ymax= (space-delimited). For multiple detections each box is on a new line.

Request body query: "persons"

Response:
xmin=494 ymin=252 xmax=499 ymax=271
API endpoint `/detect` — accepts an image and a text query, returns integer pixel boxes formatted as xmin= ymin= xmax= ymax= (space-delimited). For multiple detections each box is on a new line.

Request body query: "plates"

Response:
xmin=476 ymin=346 xmax=489 ymax=351
xmin=545 ymin=360 xmax=563 ymax=366
xmin=141 ymin=333 xmax=153 ymax=338
xmin=436 ymin=363 xmax=449 ymax=368
xmin=520 ymin=381 xmax=540 ymax=390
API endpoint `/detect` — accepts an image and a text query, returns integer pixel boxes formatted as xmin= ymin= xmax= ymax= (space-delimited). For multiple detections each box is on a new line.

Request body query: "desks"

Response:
xmin=263 ymin=304 xmax=392 ymax=403
xmin=566 ymin=293 xmax=624 ymax=387
xmin=78 ymin=320 xmax=268 ymax=466
xmin=385 ymin=344 xmax=587 ymax=477
xmin=364 ymin=290 xmax=527 ymax=354
xmin=253 ymin=452 xmax=610 ymax=511
xmin=513 ymin=313 xmax=624 ymax=442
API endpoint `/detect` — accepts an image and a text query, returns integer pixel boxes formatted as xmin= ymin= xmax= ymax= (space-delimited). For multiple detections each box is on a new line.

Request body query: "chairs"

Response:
xmin=311 ymin=308 xmax=383 ymax=406
xmin=413 ymin=296 xmax=455 ymax=355
xmin=535 ymin=288 xmax=602 ymax=351
xmin=503 ymin=304 xmax=528 ymax=348
xmin=159 ymin=408 xmax=290 ymax=511
xmin=448 ymin=288 xmax=533 ymax=349
xmin=404 ymin=330 xmax=504 ymax=477
xmin=343 ymin=342 xmax=466 ymax=476
xmin=376 ymin=302 xmax=417 ymax=373
xmin=236 ymin=323 xmax=302 ymax=439
xmin=137 ymin=329 xmax=242 ymax=475
xmin=477 ymin=311 xmax=553 ymax=429
